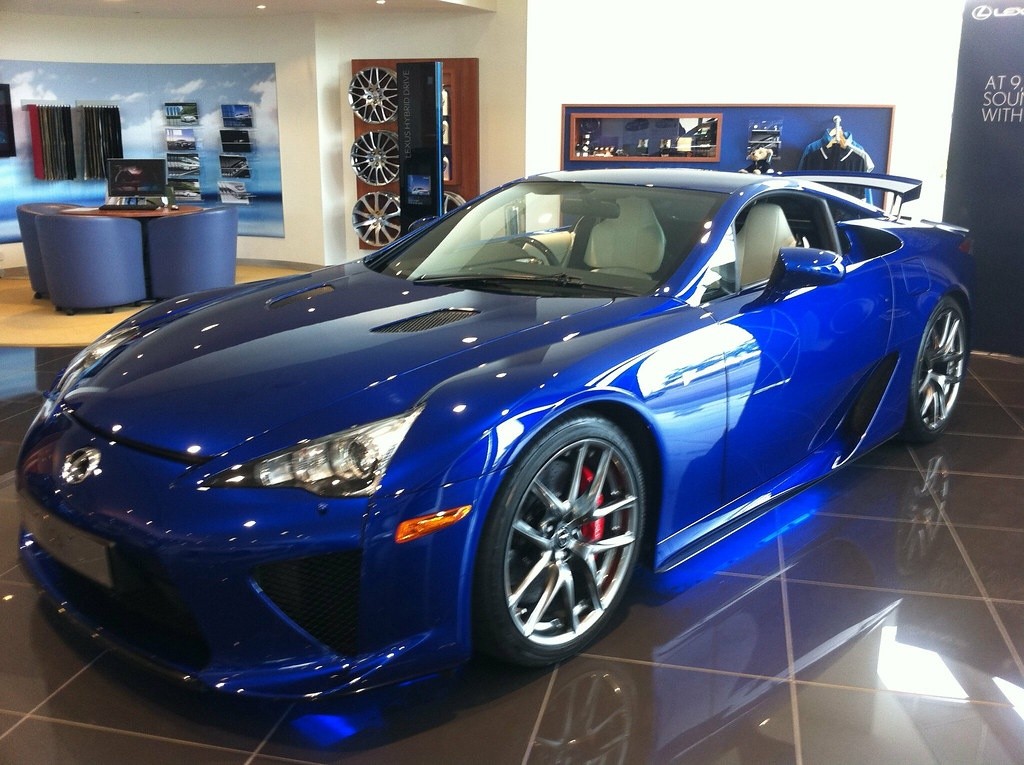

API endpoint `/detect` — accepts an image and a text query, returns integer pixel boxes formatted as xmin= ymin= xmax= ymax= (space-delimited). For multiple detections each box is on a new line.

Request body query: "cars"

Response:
xmin=235 ymin=113 xmax=248 ymax=118
xmin=9 ymin=166 xmax=984 ymax=758
xmin=181 ymin=114 xmax=196 ymax=122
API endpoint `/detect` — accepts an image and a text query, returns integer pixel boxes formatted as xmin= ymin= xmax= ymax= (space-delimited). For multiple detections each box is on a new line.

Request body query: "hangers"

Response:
xmin=825 ymin=128 xmax=846 ymax=151
xmin=828 ymin=115 xmax=849 ymax=138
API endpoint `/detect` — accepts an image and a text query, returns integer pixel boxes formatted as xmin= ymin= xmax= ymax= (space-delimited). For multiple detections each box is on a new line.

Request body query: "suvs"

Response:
xmin=175 ymin=140 xmax=188 ymax=148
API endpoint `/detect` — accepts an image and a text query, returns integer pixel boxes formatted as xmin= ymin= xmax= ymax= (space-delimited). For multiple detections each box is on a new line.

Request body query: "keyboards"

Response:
xmin=99 ymin=204 xmax=159 ymax=209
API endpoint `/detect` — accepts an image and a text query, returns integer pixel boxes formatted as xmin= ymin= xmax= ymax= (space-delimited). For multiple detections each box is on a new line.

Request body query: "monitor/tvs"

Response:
xmin=107 ymin=157 xmax=168 ymax=197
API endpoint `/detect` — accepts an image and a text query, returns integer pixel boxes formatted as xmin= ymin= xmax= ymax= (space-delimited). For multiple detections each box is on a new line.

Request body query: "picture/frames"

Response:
xmin=569 ymin=112 xmax=723 ymax=162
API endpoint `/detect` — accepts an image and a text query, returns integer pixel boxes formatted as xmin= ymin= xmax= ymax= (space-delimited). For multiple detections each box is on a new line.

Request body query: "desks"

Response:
xmin=59 ymin=205 xmax=202 ymax=218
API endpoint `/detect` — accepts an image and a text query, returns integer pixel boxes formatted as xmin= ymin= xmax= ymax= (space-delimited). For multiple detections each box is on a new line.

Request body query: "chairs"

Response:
xmin=147 ymin=204 xmax=238 ymax=303
xmin=17 ymin=203 xmax=85 ymax=300
xmin=736 ymin=202 xmax=797 ymax=288
xmin=581 ymin=193 xmax=667 ymax=281
xmin=35 ymin=214 xmax=148 ymax=316
